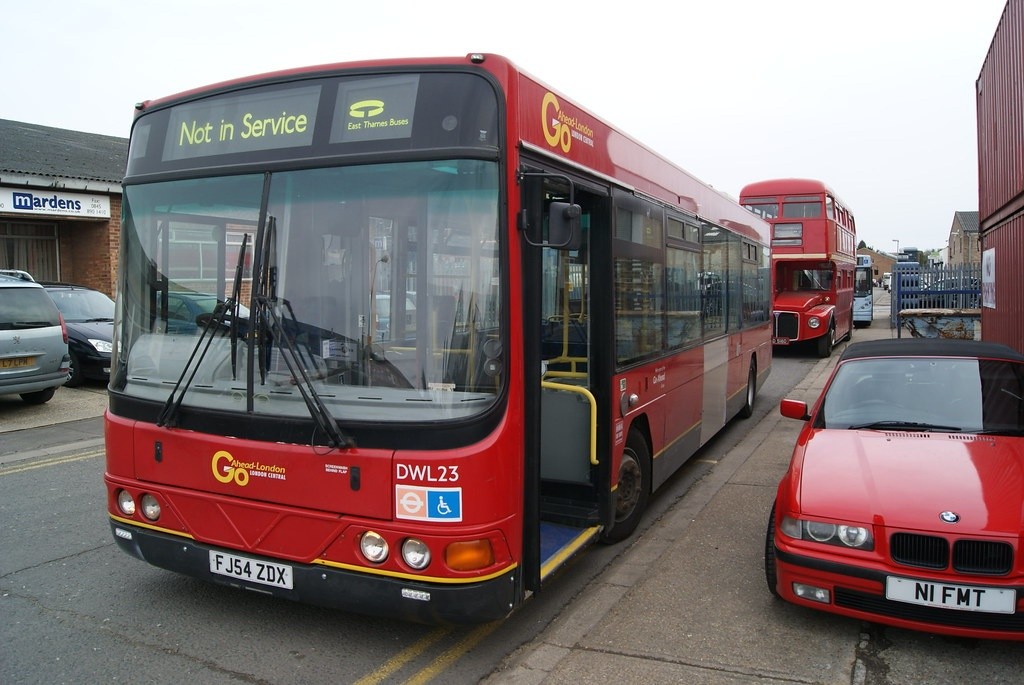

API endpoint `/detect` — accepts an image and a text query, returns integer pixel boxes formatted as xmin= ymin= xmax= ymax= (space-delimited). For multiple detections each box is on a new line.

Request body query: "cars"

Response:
xmin=882 ymin=272 xmax=891 ymax=293
xmin=920 ymin=277 xmax=982 ymax=310
xmin=156 ymin=290 xmax=260 ymax=339
xmin=42 ymin=284 xmax=121 ymax=388
xmin=762 ymin=335 xmax=1024 ymax=646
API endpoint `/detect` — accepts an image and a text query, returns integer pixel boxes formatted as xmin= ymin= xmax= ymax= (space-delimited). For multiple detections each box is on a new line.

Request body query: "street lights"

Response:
xmin=366 ymin=254 xmax=389 ymax=389
xmin=892 ymin=239 xmax=899 ymax=262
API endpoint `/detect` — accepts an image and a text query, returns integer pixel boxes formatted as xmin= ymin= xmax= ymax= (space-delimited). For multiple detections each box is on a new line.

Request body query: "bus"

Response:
xmin=98 ymin=51 xmax=777 ymax=628
xmin=736 ymin=176 xmax=858 ymax=358
xmin=811 ymin=254 xmax=879 ymax=328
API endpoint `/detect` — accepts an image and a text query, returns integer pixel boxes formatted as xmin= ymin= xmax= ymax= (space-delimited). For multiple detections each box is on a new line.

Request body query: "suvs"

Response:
xmin=0 ymin=268 xmax=73 ymax=405
